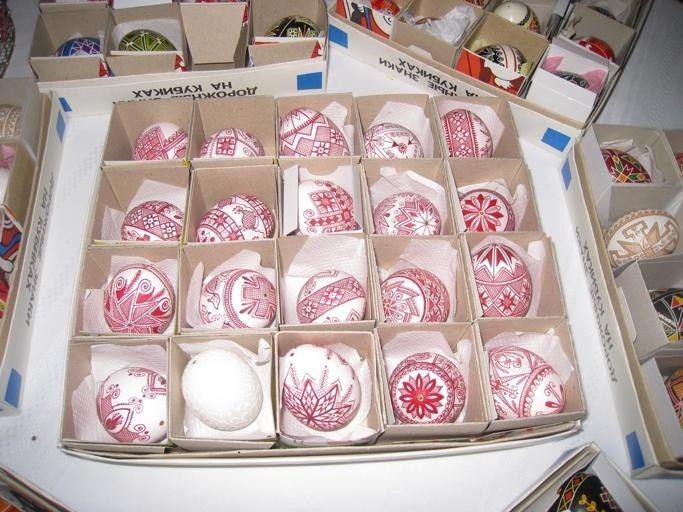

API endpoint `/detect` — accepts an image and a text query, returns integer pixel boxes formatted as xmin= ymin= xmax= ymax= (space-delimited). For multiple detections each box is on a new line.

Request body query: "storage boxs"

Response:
xmin=0 ymin=1 xmax=683 ymax=512
